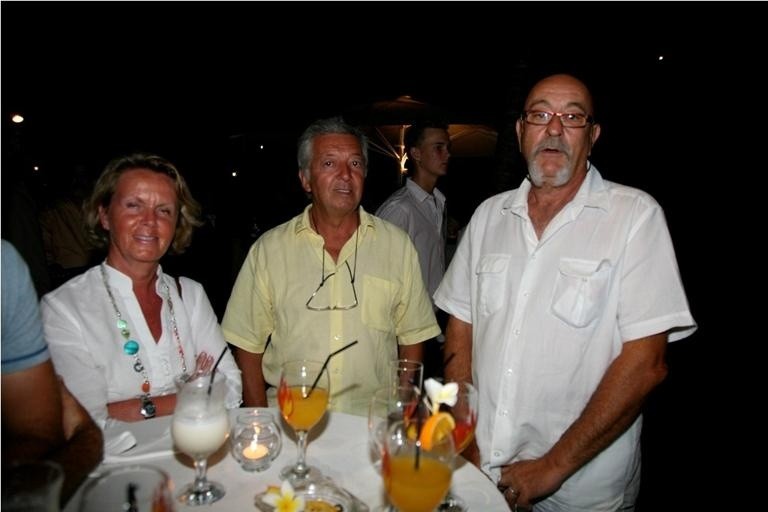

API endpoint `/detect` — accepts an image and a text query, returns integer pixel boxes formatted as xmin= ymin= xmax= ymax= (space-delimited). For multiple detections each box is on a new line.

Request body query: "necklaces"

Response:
xmin=99 ymin=262 xmax=191 ymax=420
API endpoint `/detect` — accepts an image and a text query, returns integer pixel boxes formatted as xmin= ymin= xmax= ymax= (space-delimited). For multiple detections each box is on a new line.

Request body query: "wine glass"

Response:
xmin=276 ymin=358 xmax=331 ymax=488
xmin=168 ymin=366 xmax=235 ymax=508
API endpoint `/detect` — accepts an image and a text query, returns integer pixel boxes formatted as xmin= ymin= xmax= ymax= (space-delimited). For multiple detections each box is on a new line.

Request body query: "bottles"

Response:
xmin=229 ymin=412 xmax=283 ymax=472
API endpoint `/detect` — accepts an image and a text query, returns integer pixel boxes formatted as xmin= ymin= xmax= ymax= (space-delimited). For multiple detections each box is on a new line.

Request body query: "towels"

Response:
xmin=97 ymin=416 xmax=179 ymax=467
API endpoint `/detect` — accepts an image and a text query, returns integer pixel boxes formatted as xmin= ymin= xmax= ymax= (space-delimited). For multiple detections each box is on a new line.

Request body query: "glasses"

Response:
xmin=306 ymin=259 xmax=358 ymax=311
xmin=521 ymin=108 xmax=592 ymax=129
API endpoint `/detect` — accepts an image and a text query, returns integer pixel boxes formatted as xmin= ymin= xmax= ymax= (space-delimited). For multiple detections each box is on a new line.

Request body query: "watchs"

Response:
xmin=139 ymin=394 xmax=156 ymax=418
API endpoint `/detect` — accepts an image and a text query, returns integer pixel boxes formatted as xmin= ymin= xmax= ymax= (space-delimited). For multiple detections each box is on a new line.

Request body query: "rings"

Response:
xmin=509 ymin=486 xmax=519 ymax=497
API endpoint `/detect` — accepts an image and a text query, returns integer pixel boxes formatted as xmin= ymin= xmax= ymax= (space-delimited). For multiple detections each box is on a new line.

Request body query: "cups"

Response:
xmin=78 ymin=461 xmax=175 ymax=512
xmin=370 ymin=416 xmax=457 ymax=512
xmin=426 ymin=377 xmax=479 ymax=459
xmin=366 ymin=358 xmax=425 ymax=483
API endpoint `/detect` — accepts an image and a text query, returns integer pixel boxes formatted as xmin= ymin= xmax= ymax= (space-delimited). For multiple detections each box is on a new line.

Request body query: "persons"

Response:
xmin=371 ymin=116 xmax=451 ymax=381
xmin=432 ymin=72 xmax=701 ymax=512
xmin=219 ymin=117 xmax=443 ymax=418
xmin=1 ymin=237 xmax=64 ymax=466
xmin=38 ymin=149 xmax=243 ymax=468
